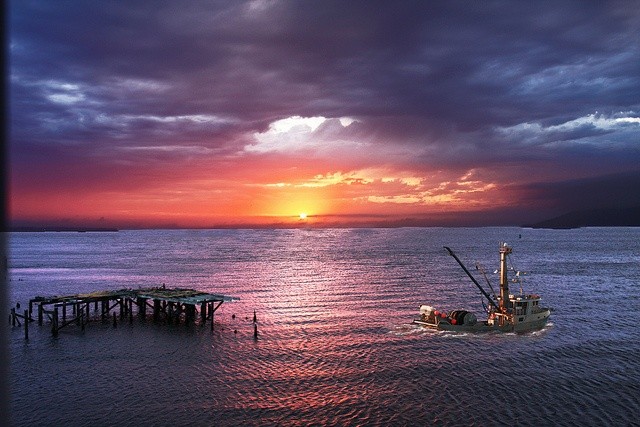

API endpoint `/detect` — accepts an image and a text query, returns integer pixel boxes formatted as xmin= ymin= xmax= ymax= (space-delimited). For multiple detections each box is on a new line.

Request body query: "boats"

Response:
xmin=412 ymin=241 xmax=551 ymax=333
